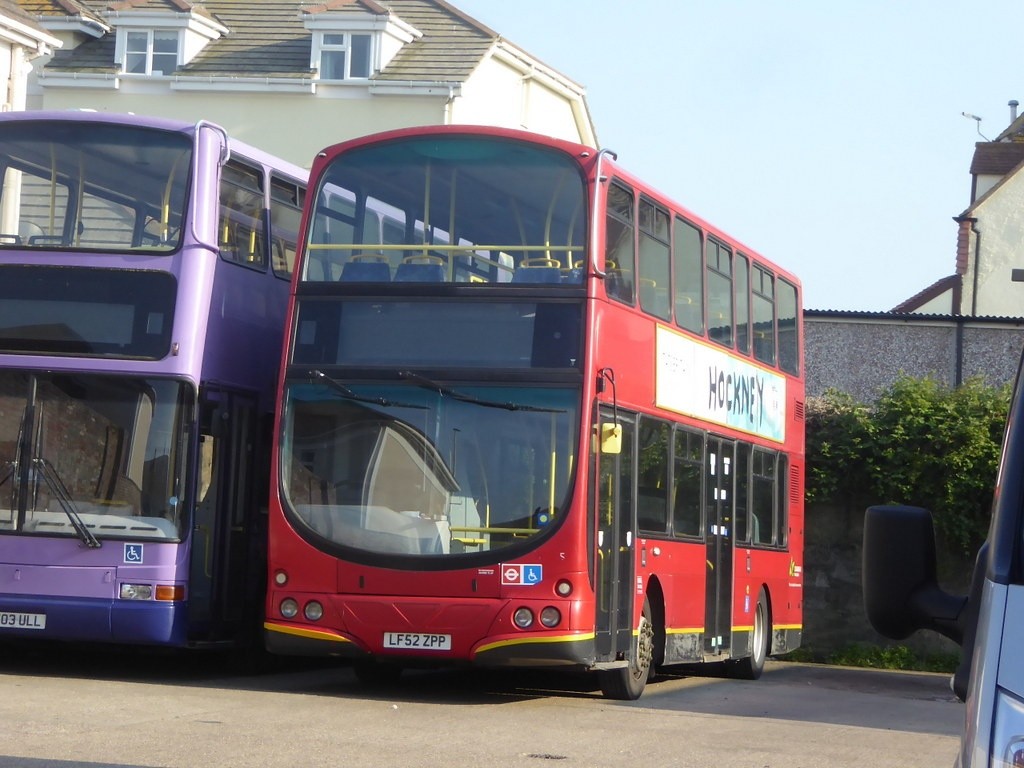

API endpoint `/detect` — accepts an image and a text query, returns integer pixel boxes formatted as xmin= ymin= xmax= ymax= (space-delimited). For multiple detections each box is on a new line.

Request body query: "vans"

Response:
xmin=859 ymin=349 xmax=1024 ymax=768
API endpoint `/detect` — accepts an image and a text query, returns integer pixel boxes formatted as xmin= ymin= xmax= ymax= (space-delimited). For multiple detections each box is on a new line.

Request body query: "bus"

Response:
xmin=257 ymin=120 xmax=809 ymax=703
xmin=0 ymin=106 xmax=525 ymax=672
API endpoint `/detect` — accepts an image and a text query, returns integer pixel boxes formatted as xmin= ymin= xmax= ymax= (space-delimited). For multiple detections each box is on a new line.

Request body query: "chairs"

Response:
xmin=337 ymin=254 xmax=445 ymax=283
xmin=0 ymin=234 xmax=62 ymax=246
xmin=512 ymin=256 xmax=774 ymax=364
